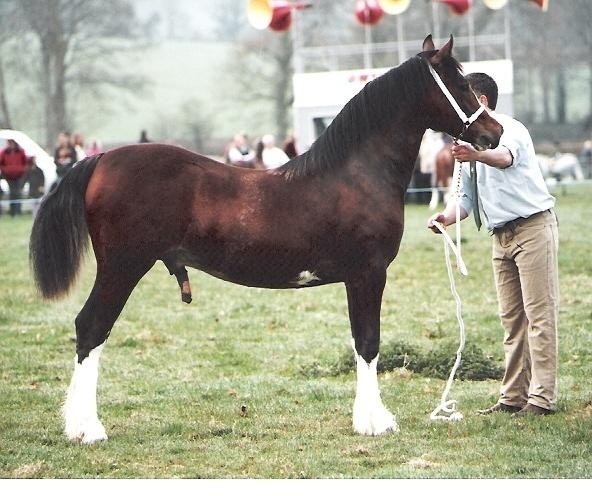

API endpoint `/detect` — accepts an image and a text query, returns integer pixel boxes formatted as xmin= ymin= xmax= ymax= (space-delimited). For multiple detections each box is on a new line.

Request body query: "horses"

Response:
xmin=27 ymin=32 xmax=504 ymax=448
xmin=435 ymin=142 xmax=456 ymax=188
xmin=536 ymin=150 xmax=582 ymax=185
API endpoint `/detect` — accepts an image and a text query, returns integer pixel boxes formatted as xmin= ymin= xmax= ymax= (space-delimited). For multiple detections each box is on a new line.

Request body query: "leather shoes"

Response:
xmin=477 ymin=403 xmax=521 ymax=414
xmin=510 ymin=402 xmax=556 ymax=418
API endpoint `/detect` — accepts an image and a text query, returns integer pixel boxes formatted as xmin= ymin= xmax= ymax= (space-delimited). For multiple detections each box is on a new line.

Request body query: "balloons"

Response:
xmin=244 ymin=0 xmax=509 ymax=30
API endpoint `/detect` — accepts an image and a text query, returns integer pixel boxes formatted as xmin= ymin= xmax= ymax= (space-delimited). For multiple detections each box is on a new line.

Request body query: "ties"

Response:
xmin=469 ymin=161 xmax=482 ymax=231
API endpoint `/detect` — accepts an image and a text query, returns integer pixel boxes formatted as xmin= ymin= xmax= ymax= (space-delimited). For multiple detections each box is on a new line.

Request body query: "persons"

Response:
xmin=426 ymin=72 xmax=559 ymax=420
xmin=1 ymin=130 xmax=104 ymax=217
xmin=223 ymin=132 xmax=300 ymax=170
